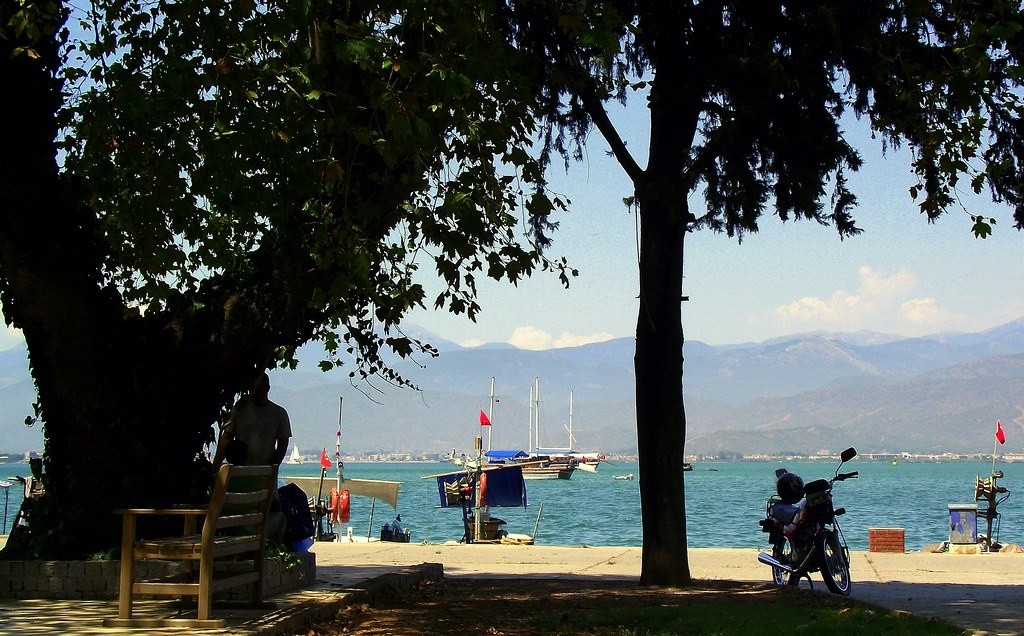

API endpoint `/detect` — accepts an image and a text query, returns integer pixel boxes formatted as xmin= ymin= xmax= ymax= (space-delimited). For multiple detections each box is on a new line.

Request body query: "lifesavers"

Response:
xmin=341 ymin=489 xmax=351 ymax=524
xmin=329 ymin=488 xmax=337 ymax=524
xmin=479 ymin=472 xmax=487 ymax=507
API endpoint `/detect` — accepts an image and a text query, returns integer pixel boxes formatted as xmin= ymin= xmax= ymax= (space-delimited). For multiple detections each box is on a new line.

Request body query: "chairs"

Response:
xmin=103 ymin=464 xmax=279 ymax=627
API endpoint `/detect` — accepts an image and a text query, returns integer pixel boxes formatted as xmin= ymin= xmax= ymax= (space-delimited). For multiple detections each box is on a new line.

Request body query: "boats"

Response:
xmin=931 ymin=420 xmax=1011 ymax=554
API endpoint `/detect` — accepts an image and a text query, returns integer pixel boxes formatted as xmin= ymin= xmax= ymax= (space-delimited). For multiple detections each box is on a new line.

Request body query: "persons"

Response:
xmin=211 ymin=373 xmax=291 ymax=544
xmin=582 ymin=456 xmax=586 ymax=464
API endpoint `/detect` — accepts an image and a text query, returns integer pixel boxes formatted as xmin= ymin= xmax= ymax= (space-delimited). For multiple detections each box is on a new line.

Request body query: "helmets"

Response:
xmin=775 ymin=473 xmax=805 ymax=504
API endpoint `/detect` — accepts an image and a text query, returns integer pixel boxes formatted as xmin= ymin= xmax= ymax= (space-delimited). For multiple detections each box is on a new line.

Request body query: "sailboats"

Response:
xmin=421 ymin=435 xmax=549 ymax=545
xmin=450 ymin=376 xmax=579 ymax=481
xmin=528 ymin=377 xmax=606 ymax=472
xmin=287 ymin=443 xmax=303 ymax=465
xmin=278 ymin=397 xmax=405 ymax=542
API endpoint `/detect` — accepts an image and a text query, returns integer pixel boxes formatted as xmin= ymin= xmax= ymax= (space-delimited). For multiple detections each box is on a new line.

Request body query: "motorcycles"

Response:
xmin=757 ymin=447 xmax=858 ymax=595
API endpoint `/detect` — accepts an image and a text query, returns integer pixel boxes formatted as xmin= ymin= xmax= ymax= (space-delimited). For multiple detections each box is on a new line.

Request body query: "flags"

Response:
xmin=480 ymin=411 xmax=491 ymax=426
xmin=321 ymin=451 xmax=332 ymax=468
xmin=996 ymin=422 xmax=1006 ymax=444
